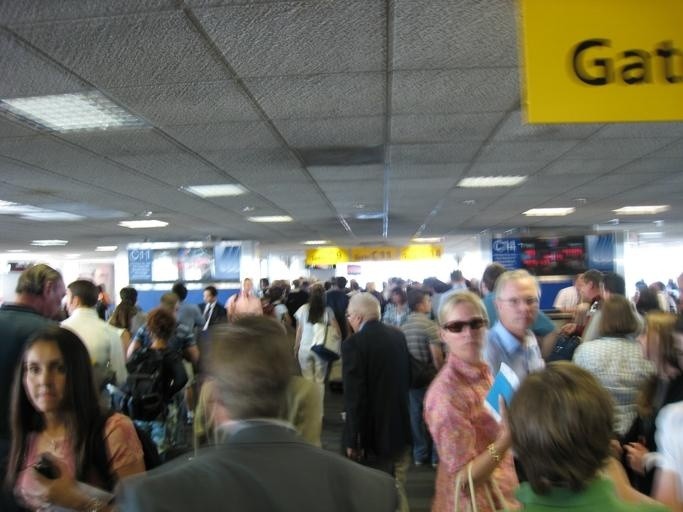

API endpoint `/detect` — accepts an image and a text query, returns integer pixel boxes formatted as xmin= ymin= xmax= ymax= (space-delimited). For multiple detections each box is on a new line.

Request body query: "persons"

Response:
xmin=2 ymin=260 xmax=682 ymax=511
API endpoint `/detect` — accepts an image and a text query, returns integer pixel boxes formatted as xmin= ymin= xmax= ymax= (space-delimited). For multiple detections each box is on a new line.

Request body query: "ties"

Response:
xmin=203 ymin=306 xmax=211 ymax=320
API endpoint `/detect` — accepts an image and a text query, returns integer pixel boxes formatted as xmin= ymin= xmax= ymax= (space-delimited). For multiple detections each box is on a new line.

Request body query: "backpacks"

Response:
xmin=121 ymin=346 xmax=180 ymax=423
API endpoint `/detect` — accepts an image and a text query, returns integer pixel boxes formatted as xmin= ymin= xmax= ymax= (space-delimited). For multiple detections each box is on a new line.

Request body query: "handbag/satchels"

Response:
xmin=310 ymin=311 xmax=342 ymax=361
xmin=549 ymin=332 xmax=580 ymax=362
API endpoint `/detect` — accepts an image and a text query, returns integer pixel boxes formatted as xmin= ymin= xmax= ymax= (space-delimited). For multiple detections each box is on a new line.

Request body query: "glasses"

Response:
xmin=345 ymin=313 xmax=351 ymax=319
xmin=444 ymin=317 xmax=489 ymax=332
